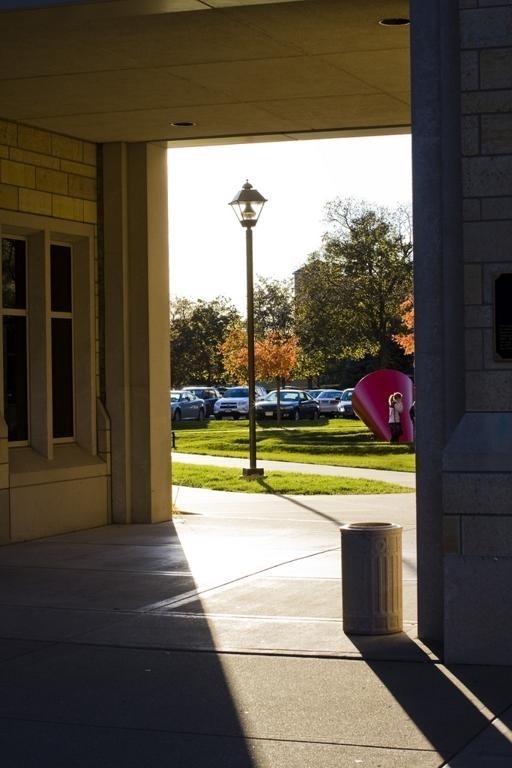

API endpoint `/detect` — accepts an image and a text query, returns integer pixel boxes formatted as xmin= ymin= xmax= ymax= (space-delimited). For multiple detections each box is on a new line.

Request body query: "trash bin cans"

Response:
xmin=339 ymin=522 xmax=403 ymax=635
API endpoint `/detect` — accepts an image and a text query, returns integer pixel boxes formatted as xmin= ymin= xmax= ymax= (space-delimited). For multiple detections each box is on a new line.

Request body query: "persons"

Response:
xmin=388 ymin=392 xmax=403 ymax=442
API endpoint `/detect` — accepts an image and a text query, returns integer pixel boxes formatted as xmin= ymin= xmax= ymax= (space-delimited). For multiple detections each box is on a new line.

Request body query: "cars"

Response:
xmin=170 ymin=384 xmax=359 ymax=426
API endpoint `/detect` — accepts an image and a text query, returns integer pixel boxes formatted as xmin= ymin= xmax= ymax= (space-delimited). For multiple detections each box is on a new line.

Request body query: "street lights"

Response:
xmin=228 ymin=179 xmax=268 ymax=477
